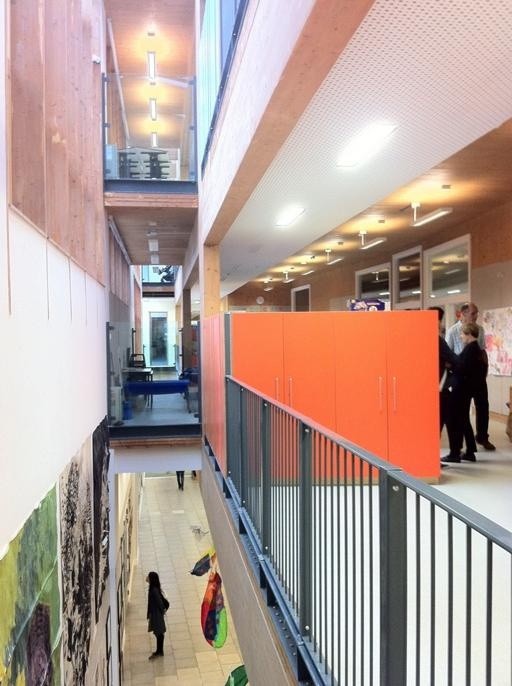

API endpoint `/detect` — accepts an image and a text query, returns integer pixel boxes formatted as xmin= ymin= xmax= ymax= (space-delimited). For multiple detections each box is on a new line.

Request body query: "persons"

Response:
xmin=446 ymin=301 xmax=496 ymax=450
xmin=428 ymin=306 xmax=458 ymax=467
xmin=146 ymin=571 xmax=170 ymax=660
xmin=440 ymin=322 xmax=483 ymax=462
xmin=176 ymin=471 xmax=185 ymax=492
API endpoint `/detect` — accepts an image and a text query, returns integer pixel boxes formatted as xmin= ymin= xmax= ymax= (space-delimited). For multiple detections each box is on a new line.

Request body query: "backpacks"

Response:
xmin=161 ymin=598 xmax=169 ymax=610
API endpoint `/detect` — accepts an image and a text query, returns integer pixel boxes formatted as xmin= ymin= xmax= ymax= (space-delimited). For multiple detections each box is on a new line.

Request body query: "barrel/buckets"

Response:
xmin=123 ymin=399 xmax=133 ymax=420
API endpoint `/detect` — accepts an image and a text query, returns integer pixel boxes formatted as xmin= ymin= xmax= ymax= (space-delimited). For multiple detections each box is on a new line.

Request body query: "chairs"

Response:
xmin=128 ymin=354 xmax=146 ymax=401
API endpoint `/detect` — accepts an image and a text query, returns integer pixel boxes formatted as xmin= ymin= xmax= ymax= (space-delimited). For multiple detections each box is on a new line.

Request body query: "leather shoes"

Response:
xmin=476 ymin=433 xmax=496 ymax=450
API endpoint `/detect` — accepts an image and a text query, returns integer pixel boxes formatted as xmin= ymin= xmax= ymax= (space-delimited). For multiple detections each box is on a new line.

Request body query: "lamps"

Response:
xmin=359 ymin=237 xmax=385 ymax=249
xmin=410 ymin=206 xmax=452 ymax=227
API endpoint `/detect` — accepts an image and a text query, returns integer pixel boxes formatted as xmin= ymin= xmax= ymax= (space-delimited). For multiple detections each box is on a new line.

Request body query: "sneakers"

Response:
xmin=440 ymin=453 xmax=475 ymax=463
xmin=149 ymin=652 xmax=163 ymax=660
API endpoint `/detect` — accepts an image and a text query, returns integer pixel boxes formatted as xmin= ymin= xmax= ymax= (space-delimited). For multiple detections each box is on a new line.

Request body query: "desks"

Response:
xmin=125 ymin=379 xmax=193 ymax=413
